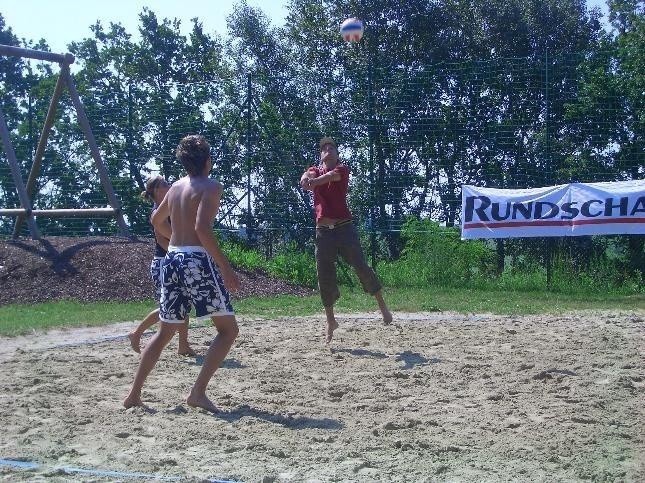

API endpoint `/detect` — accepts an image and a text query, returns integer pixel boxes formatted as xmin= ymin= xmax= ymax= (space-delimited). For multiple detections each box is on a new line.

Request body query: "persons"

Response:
xmin=122 ymin=132 xmax=241 ymax=415
xmin=127 ymin=172 xmax=195 ymax=358
xmin=299 ymin=135 xmax=395 ymax=345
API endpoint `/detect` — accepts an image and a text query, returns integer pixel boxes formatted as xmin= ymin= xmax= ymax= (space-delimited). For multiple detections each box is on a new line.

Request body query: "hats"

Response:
xmin=318 ymin=135 xmax=340 ymax=150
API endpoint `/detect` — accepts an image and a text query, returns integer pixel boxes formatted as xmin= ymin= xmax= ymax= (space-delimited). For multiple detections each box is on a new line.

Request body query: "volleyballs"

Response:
xmin=340 ymin=16 xmax=364 ymax=41
